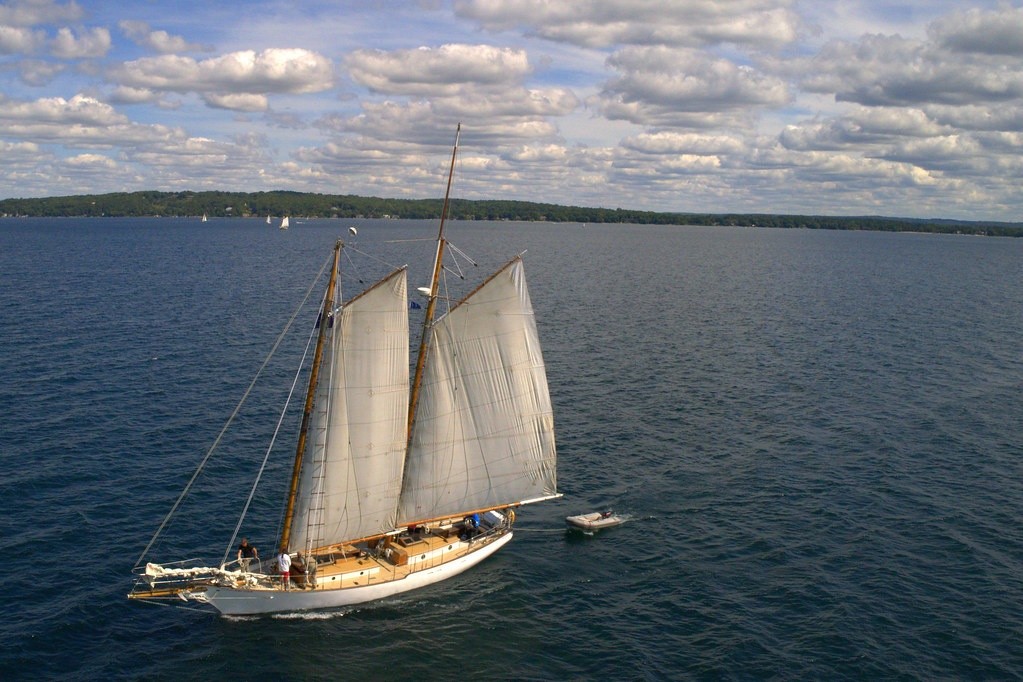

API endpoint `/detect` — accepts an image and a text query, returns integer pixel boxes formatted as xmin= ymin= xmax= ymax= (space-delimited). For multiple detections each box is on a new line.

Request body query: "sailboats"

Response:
xmin=126 ymin=119 xmax=566 ymax=618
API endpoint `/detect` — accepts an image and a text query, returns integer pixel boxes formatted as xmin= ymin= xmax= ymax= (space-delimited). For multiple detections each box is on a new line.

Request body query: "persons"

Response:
xmin=297 ymin=551 xmax=318 ymax=588
xmin=237 ymin=538 xmax=257 ymax=572
xmin=277 ymin=548 xmax=291 ymax=591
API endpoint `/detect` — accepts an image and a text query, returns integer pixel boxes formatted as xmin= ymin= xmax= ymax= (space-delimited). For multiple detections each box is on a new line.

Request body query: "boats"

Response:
xmin=565 ymin=512 xmax=624 ymax=529
